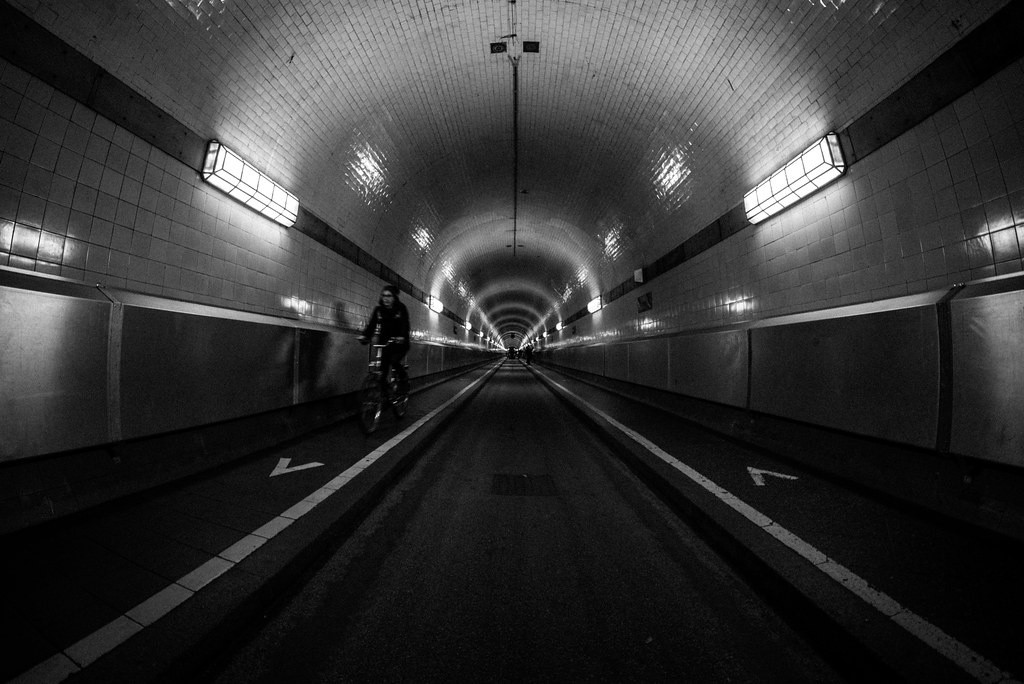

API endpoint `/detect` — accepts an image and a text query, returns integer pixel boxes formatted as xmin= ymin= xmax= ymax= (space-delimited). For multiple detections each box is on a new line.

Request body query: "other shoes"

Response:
xmin=398 ymin=375 xmax=409 ymax=392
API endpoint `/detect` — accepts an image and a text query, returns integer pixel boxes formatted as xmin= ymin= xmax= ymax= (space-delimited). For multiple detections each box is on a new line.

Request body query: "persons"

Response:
xmin=526 ymin=346 xmax=533 ymax=365
xmin=353 ymin=285 xmax=412 ymax=394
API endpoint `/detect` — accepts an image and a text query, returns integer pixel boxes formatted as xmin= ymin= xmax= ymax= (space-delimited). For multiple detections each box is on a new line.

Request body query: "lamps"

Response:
xmin=744 ymin=131 xmax=848 ymax=224
xmin=201 ymin=138 xmax=300 ymax=227
xmin=428 ymin=293 xmax=443 ymax=313
xmin=587 ymin=296 xmax=602 ymax=315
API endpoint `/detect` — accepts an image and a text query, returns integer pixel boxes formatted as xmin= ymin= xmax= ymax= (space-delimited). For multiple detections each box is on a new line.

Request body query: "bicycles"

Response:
xmin=355 ymin=337 xmax=412 ymax=437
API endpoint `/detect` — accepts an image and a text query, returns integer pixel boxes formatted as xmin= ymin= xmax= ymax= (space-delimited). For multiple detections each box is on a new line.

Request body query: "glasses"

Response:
xmin=380 ymin=293 xmax=394 ymax=298
